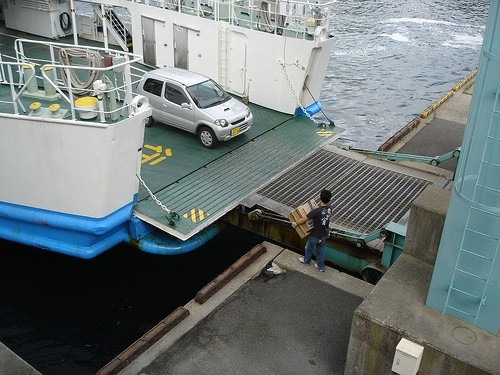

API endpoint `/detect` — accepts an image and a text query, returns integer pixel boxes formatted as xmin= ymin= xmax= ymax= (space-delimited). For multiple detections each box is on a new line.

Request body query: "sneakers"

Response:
xmin=314 ymin=262 xmax=325 ymax=272
xmin=298 ymin=257 xmax=310 ymax=266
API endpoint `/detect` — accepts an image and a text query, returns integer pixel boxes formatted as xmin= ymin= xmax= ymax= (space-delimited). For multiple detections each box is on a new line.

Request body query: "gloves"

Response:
xmin=292 ymin=222 xmax=298 ymax=228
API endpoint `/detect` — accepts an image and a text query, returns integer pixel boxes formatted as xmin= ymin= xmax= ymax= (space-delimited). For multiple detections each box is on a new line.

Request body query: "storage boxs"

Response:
xmin=289 ymin=199 xmax=320 ymax=238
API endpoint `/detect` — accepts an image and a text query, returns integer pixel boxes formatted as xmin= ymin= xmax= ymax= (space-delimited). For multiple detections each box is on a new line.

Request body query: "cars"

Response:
xmin=135 ymin=67 xmax=254 ymax=149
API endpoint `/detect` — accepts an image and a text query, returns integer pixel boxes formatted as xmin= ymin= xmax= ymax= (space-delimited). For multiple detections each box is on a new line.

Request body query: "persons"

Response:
xmin=292 ymin=190 xmax=332 ymax=272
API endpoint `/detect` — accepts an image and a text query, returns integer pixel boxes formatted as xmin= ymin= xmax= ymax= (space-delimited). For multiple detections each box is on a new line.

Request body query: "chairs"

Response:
xmin=202 ymin=89 xmax=219 ymax=100
